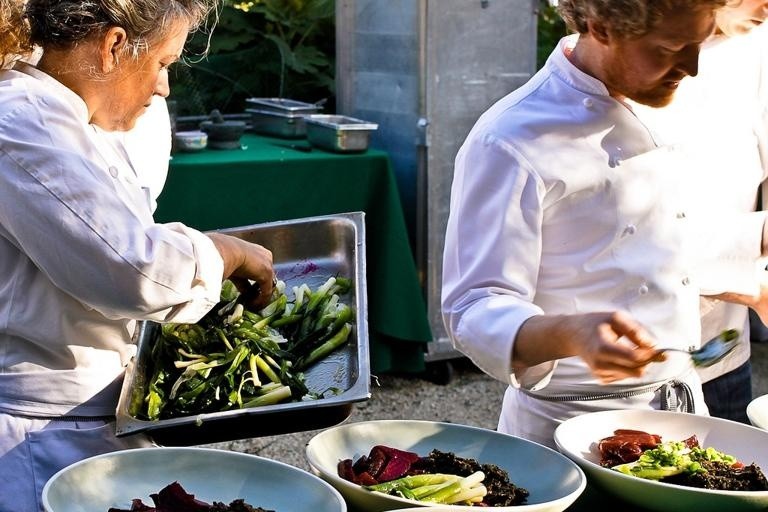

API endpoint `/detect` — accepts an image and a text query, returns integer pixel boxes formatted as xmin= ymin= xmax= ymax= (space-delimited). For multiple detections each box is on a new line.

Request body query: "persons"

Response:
xmin=667 ymin=0 xmax=768 ymax=425
xmin=440 ymin=0 xmax=768 ymax=450
xmin=1 ymin=0 xmax=277 ymax=512
xmin=0 ymin=0 xmax=173 ymax=213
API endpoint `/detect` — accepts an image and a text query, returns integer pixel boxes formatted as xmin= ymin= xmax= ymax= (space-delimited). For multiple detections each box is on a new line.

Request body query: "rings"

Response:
xmin=273 ymin=278 xmax=276 ymax=287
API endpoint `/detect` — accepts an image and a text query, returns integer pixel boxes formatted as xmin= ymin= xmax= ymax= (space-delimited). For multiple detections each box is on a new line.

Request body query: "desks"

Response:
xmin=153 ymin=128 xmax=392 ymax=388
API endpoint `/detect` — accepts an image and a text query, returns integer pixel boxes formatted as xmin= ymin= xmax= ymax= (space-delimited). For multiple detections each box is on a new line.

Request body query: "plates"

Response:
xmin=306 ymin=420 xmax=588 ymax=512
xmin=553 ymin=409 xmax=767 ymax=511
xmin=40 ymin=446 xmax=347 ymax=512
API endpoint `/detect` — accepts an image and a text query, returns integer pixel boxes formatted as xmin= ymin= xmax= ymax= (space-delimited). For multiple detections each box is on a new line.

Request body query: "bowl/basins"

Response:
xmin=175 ymin=131 xmax=209 ymax=154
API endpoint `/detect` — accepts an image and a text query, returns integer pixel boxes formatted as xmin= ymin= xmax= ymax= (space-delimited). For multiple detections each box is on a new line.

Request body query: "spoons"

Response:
xmin=658 ymin=329 xmax=740 ymax=369
